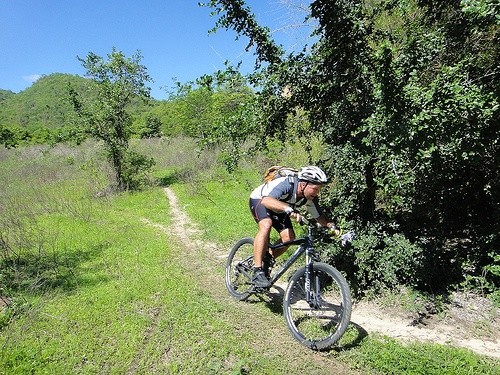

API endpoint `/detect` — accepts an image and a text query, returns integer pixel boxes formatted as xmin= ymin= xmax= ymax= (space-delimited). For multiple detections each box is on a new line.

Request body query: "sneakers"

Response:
xmin=252 ymin=270 xmax=272 ymax=288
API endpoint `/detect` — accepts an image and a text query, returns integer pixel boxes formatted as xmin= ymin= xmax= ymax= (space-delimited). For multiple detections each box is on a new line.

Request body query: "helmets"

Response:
xmin=298 ymin=166 xmax=328 ymax=184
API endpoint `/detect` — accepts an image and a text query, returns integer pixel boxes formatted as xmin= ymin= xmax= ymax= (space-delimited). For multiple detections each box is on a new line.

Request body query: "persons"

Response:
xmin=248 ymin=165 xmax=338 ymax=288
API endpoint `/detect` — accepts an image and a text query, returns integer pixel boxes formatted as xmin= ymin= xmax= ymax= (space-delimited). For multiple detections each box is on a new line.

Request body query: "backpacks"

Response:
xmin=262 ymin=165 xmax=298 ymax=185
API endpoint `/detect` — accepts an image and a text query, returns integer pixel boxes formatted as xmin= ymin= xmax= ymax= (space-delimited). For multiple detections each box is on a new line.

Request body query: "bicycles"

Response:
xmin=224 ymin=210 xmax=353 ymax=352
